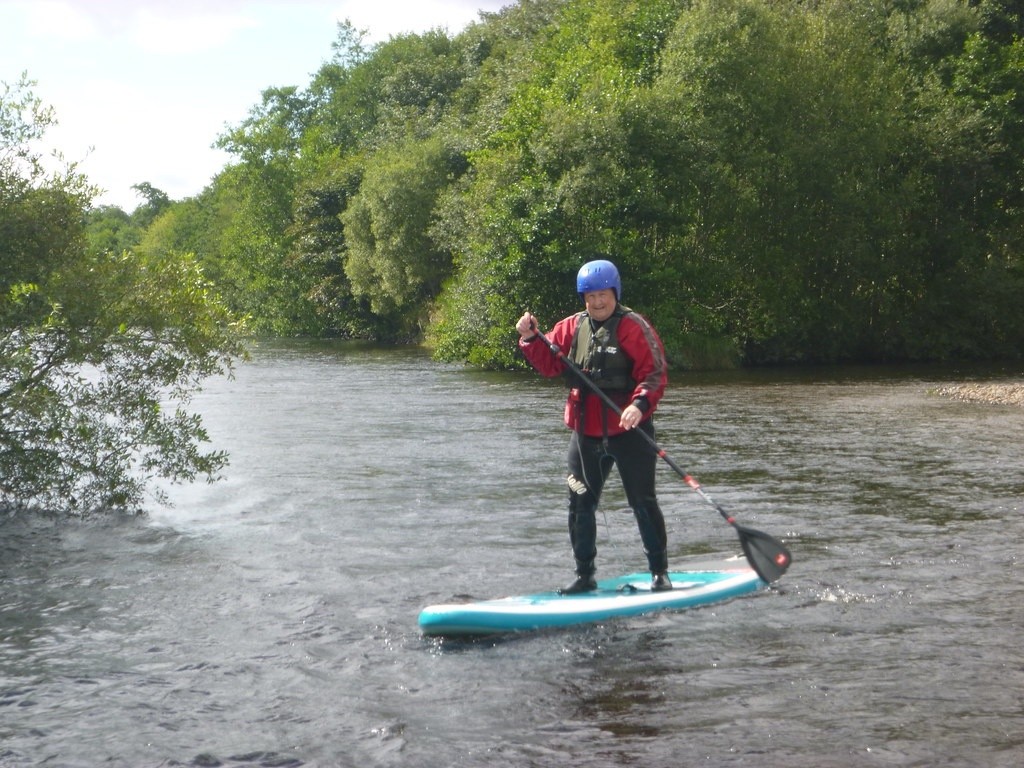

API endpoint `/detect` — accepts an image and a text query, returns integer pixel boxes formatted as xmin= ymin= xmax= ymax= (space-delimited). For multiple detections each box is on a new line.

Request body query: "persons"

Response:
xmin=516 ymin=260 xmax=673 ymax=597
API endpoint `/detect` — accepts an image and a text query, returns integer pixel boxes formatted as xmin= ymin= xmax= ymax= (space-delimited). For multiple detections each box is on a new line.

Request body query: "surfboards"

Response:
xmin=418 ymin=554 xmax=767 ymax=637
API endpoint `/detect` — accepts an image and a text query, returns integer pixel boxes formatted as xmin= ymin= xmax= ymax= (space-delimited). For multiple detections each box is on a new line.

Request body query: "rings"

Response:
xmin=632 ymin=416 xmax=636 ymax=420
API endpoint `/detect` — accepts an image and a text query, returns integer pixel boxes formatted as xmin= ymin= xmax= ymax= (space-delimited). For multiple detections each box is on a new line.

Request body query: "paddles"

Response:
xmin=529 ymin=318 xmax=793 ymax=587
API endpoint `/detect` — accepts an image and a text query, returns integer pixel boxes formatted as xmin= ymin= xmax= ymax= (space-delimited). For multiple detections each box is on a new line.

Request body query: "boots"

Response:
xmin=556 ymin=548 xmax=598 ymax=594
xmin=642 ymin=545 xmax=672 ymax=593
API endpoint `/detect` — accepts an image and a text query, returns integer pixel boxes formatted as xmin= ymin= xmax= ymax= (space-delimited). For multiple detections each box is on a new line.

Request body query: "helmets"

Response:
xmin=576 ymin=260 xmax=622 ymax=302
